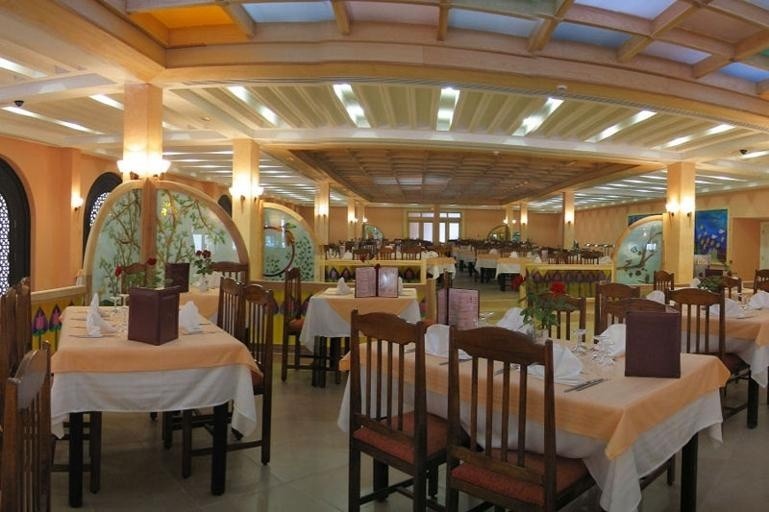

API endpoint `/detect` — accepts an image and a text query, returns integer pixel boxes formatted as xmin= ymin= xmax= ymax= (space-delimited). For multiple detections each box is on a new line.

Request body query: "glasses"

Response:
xmin=519 ymin=217 xmax=528 ymax=226
xmin=69 ymin=195 xmax=83 ymax=211
xmin=662 ymin=198 xmax=698 ymax=218
xmin=229 ymin=176 xmax=264 ymax=201
xmin=312 ymin=204 xmax=329 ymax=219
xmin=561 ymin=211 xmax=574 ymax=224
xmin=116 ymin=150 xmax=171 ymax=180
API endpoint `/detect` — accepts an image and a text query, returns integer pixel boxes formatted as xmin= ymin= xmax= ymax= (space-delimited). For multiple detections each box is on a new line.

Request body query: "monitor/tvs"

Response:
xmin=128 ymin=287 xmax=179 ymax=346
xmin=624 ymin=305 xmax=682 ymax=378
xmin=355 ymin=267 xmax=398 ymax=298
xmin=436 ymin=286 xmax=480 ymax=330
xmin=705 ymin=269 xmax=723 ymax=294
xmin=164 ymin=263 xmax=190 ymax=292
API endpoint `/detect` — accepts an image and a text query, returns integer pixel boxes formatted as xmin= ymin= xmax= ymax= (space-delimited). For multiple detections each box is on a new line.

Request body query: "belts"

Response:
xmin=478 ymin=310 xmax=494 ymax=330
xmin=569 ymin=326 xmax=591 ymax=370
xmin=736 ymin=293 xmax=749 ymax=305
xmin=118 ymin=293 xmax=129 ymax=324
xmin=756 ymin=287 xmax=765 ymax=318
xmin=109 ymin=296 xmax=126 ymax=329
xmin=591 ymin=333 xmax=615 ymax=380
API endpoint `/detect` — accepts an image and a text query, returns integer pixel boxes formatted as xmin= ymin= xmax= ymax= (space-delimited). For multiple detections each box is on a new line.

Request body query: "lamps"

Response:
xmin=282 ymin=267 xmax=349 ymax=384
xmin=165 ymin=282 xmax=274 ymax=496
xmin=593 ymin=283 xmax=640 ymax=343
xmin=604 ymin=297 xmax=665 ymax=335
xmin=528 ymin=292 xmax=587 ymax=342
xmin=664 ymin=285 xmax=751 ymax=423
xmin=539 ymin=248 xmax=601 ymax=264
xmin=150 ymin=276 xmax=244 ymax=440
xmin=349 ymin=309 xmax=469 ymax=512
xmin=324 ymin=238 xmax=422 ymax=260
xmin=0 ymin=340 xmax=51 ymax=512
xmin=720 ymin=275 xmax=741 ymax=301
xmin=17 ymin=277 xmax=32 ymax=353
xmin=444 ymin=324 xmax=594 ymax=512
xmin=202 ymin=262 xmax=248 ymax=284
xmin=753 ymin=270 xmax=769 ymax=293
xmin=449 ymin=239 xmax=529 ymax=257
xmin=654 ymin=270 xmax=674 ymax=291
xmin=0 ymin=288 xmax=101 ymax=493
xmin=443 ymin=268 xmax=449 ymax=288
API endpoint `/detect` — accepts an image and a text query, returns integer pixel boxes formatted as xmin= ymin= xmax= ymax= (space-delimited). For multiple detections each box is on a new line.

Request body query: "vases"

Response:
xmin=511 ymin=275 xmax=577 ymax=336
xmin=103 ymin=255 xmax=157 ymax=297
xmin=361 ymin=254 xmax=380 ymax=267
xmin=193 ymin=249 xmax=214 ymax=276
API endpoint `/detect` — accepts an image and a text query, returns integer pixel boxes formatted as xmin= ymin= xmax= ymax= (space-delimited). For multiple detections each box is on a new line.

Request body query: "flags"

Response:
xmin=199 ymin=276 xmax=208 ymax=293
xmin=534 ymin=328 xmax=548 ymax=345
